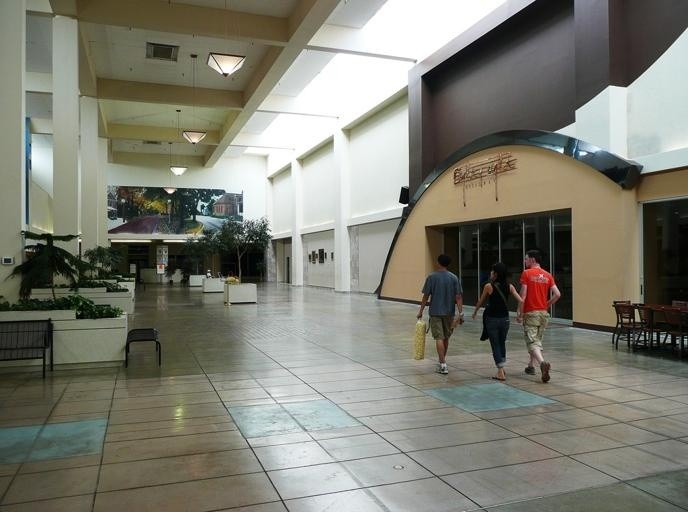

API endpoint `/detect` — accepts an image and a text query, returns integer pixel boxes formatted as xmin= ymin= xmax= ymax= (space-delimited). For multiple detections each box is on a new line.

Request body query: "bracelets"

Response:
xmin=458 ymin=312 xmax=464 ymax=316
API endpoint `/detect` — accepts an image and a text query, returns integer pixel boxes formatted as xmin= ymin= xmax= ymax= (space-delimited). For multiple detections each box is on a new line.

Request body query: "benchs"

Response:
xmin=0 ymin=317 xmax=54 ymax=378
xmin=125 ymin=327 xmax=163 ymax=368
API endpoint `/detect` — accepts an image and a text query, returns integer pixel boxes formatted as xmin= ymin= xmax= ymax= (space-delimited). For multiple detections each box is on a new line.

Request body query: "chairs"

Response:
xmin=612 ymin=300 xmax=688 ymax=360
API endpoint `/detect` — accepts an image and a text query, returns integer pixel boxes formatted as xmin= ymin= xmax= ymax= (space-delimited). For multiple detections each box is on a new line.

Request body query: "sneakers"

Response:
xmin=435 ymin=365 xmax=449 ymax=374
xmin=525 ymin=365 xmax=537 ymax=375
xmin=540 ymin=360 xmax=551 ymax=382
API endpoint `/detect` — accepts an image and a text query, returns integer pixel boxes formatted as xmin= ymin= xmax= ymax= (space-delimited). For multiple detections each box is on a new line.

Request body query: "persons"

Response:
xmin=515 ymin=249 xmax=563 ymax=384
xmin=471 ymin=262 xmax=525 ymax=380
xmin=417 ymin=253 xmax=465 ymax=376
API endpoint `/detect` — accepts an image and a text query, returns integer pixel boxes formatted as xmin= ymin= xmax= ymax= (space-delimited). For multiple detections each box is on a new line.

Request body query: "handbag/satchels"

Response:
xmin=415 ymin=317 xmax=426 ymax=360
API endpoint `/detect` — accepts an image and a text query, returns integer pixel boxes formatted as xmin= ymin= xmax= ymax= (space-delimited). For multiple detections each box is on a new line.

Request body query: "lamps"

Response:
xmin=170 ymin=166 xmax=188 ymax=176
xmin=207 ymin=52 xmax=246 ymax=78
xmin=162 ymin=187 xmax=178 ymax=195
xmin=181 ymin=130 xmax=207 ymax=146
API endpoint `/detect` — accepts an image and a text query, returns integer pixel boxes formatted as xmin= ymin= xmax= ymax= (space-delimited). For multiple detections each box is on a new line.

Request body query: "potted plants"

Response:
xmin=165 ymin=216 xmax=273 ymax=306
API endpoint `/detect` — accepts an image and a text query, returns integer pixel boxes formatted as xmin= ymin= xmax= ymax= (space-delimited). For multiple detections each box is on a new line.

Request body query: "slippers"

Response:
xmin=492 ymin=374 xmax=506 ymax=381
